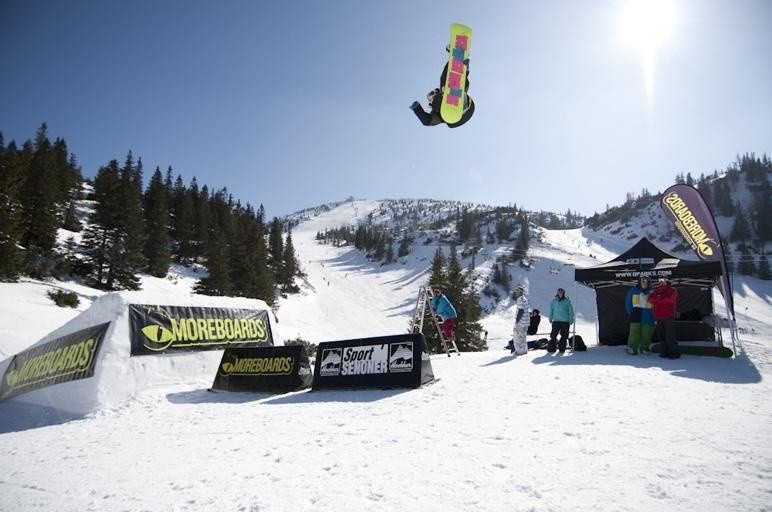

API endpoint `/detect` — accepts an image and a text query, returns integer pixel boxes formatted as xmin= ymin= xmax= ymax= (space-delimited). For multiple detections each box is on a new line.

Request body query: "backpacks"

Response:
xmin=569 ymin=335 xmax=587 ymax=351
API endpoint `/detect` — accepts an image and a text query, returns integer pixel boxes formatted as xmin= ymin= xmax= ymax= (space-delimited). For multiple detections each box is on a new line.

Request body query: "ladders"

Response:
xmin=410 ymin=285 xmax=460 ymax=357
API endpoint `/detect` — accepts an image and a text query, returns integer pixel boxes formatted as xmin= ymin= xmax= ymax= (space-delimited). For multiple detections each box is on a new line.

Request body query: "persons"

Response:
xmin=430 ymin=288 xmax=458 ymax=341
xmin=511 ymin=286 xmax=531 ymax=355
xmin=646 ymin=276 xmax=681 ymax=359
xmin=623 ymin=274 xmax=660 ymax=356
xmin=526 ymin=308 xmax=541 ymax=334
xmin=544 ymin=287 xmax=575 ymax=353
xmin=409 ymin=44 xmax=475 ymax=129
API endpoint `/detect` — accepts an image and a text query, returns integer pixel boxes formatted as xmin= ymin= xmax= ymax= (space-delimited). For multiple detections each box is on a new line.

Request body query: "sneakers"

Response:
xmin=626 ymin=346 xmax=649 ymax=356
xmin=659 ymin=350 xmax=681 ymax=360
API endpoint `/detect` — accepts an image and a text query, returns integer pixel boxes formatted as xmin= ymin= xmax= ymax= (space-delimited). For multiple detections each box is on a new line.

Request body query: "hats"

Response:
xmin=638 ymin=274 xmax=652 ymax=284
xmin=514 ymin=286 xmax=524 ymax=294
xmin=658 ymin=275 xmax=674 ymax=283
xmin=533 ymin=309 xmax=539 ymax=315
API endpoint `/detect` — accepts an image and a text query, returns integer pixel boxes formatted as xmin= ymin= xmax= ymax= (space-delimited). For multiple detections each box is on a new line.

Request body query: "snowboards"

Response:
xmin=652 ymin=343 xmax=733 ymax=358
xmin=440 ymin=23 xmax=473 ymax=124
